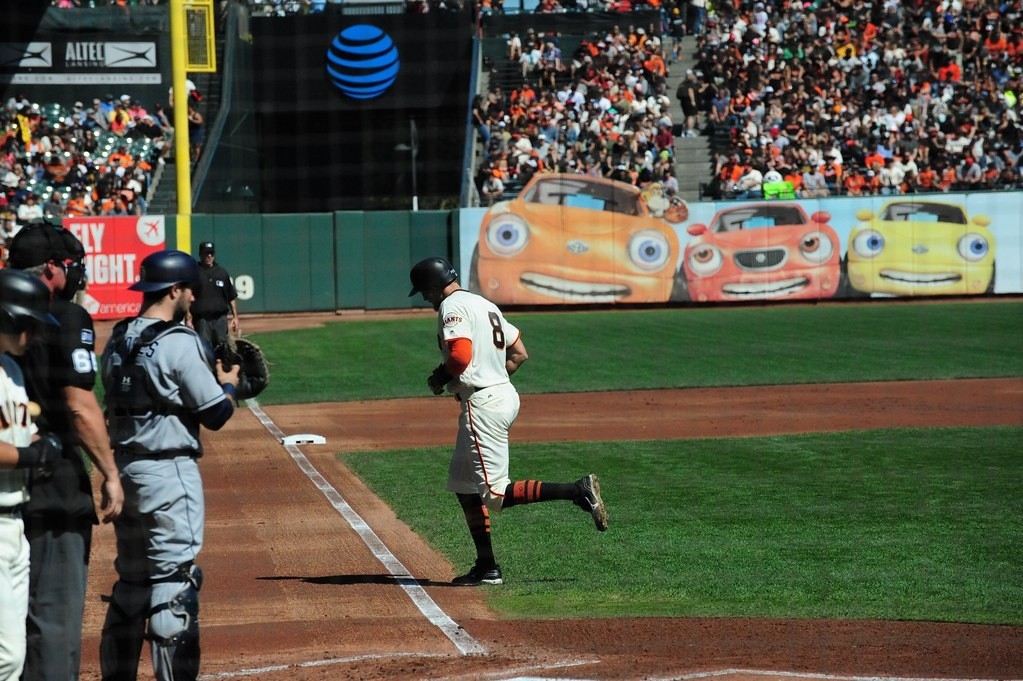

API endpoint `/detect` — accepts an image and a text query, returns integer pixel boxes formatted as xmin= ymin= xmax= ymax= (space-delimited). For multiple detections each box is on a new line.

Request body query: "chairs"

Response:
xmin=28 ymin=101 xmax=155 ymax=217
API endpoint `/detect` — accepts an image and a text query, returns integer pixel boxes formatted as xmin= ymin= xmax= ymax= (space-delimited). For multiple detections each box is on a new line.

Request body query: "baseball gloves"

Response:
xmin=213 ymin=338 xmax=271 ymax=399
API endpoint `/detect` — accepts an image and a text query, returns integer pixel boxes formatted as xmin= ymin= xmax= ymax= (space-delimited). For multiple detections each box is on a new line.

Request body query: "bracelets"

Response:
xmin=223 ymin=382 xmax=236 ymax=397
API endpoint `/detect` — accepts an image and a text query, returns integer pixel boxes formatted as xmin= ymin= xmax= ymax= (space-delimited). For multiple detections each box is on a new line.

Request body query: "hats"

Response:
xmin=105 ymin=93 xmax=114 ymax=100
xmin=10 ymin=223 xmax=86 ymax=269
xmin=119 ymin=93 xmax=132 ymax=102
xmin=199 ymin=241 xmax=214 ymax=254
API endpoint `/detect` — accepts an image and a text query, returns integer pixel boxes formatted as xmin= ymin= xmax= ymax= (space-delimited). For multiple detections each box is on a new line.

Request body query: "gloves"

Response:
xmin=17 ymin=432 xmax=64 ymax=479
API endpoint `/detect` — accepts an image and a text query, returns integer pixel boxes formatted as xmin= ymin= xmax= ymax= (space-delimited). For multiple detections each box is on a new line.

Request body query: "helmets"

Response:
xmin=408 ymin=256 xmax=460 ymax=300
xmin=2 ymin=267 xmax=61 ymax=328
xmin=128 ymin=250 xmax=197 ymax=292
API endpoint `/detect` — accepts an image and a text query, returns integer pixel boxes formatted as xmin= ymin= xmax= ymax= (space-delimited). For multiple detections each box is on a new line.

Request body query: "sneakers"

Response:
xmin=451 ymin=562 xmax=504 ymax=586
xmin=573 ymin=474 xmax=609 ymax=531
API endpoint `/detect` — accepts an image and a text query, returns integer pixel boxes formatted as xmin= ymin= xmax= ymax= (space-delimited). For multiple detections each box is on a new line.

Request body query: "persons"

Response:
xmin=0 ymin=0 xmax=1023 ymax=267
xmin=407 ymin=256 xmax=608 ymax=586
xmin=0 ymin=219 xmax=240 ymax=681
